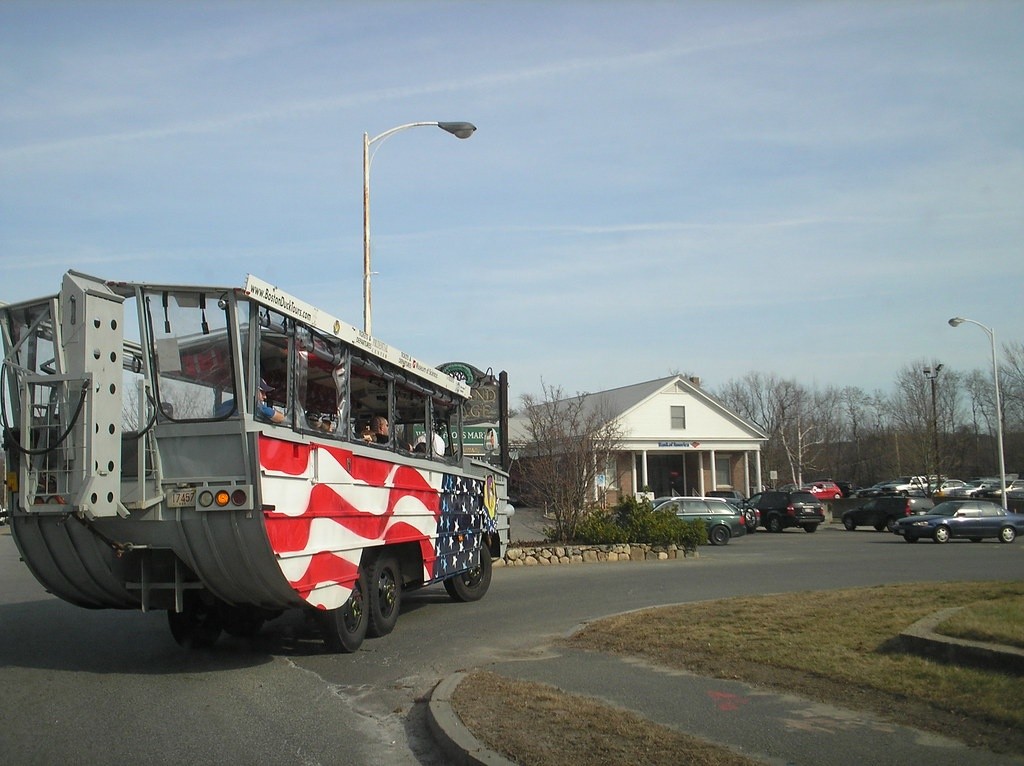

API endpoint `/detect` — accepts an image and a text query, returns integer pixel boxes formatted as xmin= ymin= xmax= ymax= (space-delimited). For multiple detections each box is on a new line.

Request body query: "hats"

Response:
xmin=322 ymin=415 xmax=332 ymax=425
xmin=260 ymin=377 xmax=276 ymax=393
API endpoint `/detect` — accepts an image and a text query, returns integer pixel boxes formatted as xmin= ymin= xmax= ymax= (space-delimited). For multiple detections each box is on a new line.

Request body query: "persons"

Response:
xmin=214 ymin=378 xmax=287 ymax=424
xmin=305 ymin=404 xmax=445 ymax=457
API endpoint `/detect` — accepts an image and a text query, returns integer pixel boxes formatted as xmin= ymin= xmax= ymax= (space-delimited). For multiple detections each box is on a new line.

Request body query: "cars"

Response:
xmin=840 ymin=494 xmax=936 ymax=534
xmin=893 ymin=500 xmax=1024 ymax=544
xmin=833 ymin=481 xmax=855 ymax=497
xmin=725 ymin=497 xmax=760 ymax=528
xmin=856 ymin=474 xmax=1024 ymax=508
xmin=781 ymin=483 xmax=799 ymax=492
xmin=801 ymin=481 xmax=843 ymax=500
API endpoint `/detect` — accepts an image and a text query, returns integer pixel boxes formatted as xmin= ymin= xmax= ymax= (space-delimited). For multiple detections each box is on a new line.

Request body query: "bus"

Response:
xmin=0 ymin=268 xmax=519 ymax=653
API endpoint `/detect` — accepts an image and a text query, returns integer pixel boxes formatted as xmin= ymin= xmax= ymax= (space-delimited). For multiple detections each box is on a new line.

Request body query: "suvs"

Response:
xmin=644 ymin=497 xmax=745 ymax=547
xmin=706 ymin=490 xmax=746 ymax=511
xmin=745 ymin=489 xmax=826 ymax=534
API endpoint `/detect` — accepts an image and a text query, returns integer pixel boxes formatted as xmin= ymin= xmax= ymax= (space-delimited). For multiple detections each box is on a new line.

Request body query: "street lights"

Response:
xmin=949 ymin=315 xmax=1007 ymax=511
xmin=923 ymin=364 xmax=945 ymax=493
xmin=361 ymin=120 xmax=477 ymax=337
